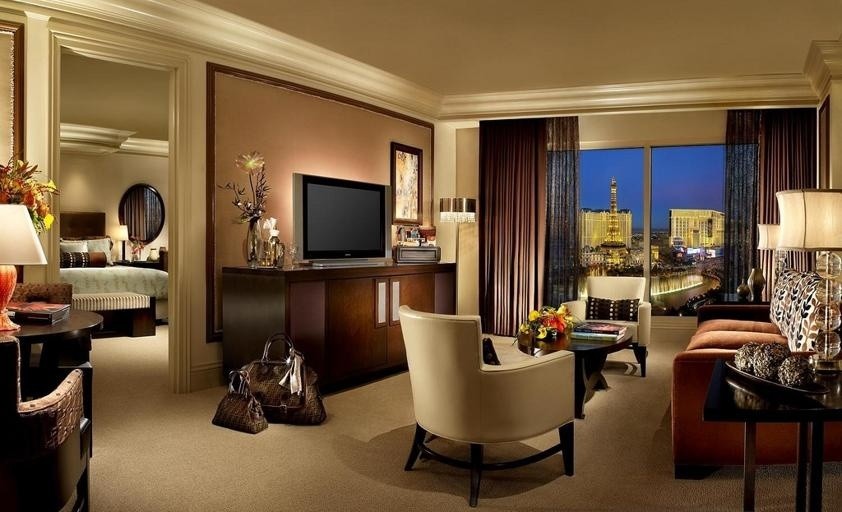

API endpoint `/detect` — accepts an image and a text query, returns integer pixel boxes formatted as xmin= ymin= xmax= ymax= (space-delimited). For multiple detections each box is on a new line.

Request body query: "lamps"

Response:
xmin=0 ymin=199 xmax=52 ymax=328
xmin=115 ymin=222 xmax=129 ymax=261
xmin=771 ymin=187 xmax=841 ymax=377
xmin=755 ymin=221 xmax=788 ymax=275
xmin=438 ymin=195 xmax=478 ymax=317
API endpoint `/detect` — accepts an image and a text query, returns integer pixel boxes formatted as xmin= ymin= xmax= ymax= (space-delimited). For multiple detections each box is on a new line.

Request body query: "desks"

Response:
xmin=707 ymin=289 xmax=771 ymax=305
xmin=518 ymin=326 xmax=634 ymax=421
xmin=698 ymin=359 xmax=842 ymax=510
xmin=1 ymin=307 xmax=108 ymax=404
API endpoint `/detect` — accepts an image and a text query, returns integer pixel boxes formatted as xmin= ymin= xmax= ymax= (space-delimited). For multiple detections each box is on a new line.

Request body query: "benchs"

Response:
xmin=70 ymin=291 xmax=159 ymax=338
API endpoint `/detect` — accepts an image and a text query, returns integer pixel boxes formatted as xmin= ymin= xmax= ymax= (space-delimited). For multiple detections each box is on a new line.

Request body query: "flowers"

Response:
xmin=217 ymin=148 xmax=274 ymax=226
xmin=1 ymin=159 xmax=56 ymax=238
xmin=514 ymin=302 xmax=578 ymax=341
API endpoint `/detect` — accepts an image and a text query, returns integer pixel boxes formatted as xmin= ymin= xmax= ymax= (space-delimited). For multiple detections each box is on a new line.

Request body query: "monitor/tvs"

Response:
xmin=292 ymin=172 xmax=393 ymax=267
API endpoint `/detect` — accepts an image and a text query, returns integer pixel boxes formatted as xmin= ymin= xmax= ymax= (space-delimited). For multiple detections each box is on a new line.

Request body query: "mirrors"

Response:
xmin=116 ymin=181 xmax=167 ymax=247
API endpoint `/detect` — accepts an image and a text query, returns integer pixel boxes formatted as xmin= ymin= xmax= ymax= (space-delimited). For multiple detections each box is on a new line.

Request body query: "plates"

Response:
xmin=723 ymin=361 xmax=830 ymax=398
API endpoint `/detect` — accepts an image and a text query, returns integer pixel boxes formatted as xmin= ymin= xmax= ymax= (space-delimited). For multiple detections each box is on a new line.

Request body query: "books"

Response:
xmin=7 ymin=304 xmax=71 ymax=325
xmin=571 ymin=323 xmax=627 ymax=342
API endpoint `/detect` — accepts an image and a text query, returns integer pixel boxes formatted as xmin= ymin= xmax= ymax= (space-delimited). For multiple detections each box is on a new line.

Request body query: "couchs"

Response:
xmin=667 ymin=266 xmax=841 ymax=479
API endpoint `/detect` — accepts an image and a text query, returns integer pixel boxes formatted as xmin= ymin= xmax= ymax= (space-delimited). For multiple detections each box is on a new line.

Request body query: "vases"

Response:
xmin=247 ymin=219 xmax=264 ymax=270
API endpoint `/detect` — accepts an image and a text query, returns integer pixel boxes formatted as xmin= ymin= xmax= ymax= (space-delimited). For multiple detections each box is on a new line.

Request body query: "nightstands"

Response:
xmin=117 ymin=259 xmax=164 ymax=272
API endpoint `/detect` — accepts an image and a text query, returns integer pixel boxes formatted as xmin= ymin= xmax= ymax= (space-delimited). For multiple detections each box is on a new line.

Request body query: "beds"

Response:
xmin=56 ymin=208 xmax=170 ymax=326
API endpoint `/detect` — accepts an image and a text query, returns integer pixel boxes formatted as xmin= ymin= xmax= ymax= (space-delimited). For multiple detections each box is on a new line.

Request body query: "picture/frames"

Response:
xmin=388 ymin=138 xmax=426 ymax=226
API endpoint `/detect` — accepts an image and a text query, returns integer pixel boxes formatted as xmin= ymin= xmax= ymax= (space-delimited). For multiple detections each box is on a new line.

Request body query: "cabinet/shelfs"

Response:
xmin=216 ymin=257 xmax=460 ymax=399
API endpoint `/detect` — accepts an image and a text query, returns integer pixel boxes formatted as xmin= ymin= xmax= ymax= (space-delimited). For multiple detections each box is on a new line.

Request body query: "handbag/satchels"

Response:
xmin=237 ymin=332 xmax=328 ymax=426
xmin=211 ymin=368 xmax=270 ymax=434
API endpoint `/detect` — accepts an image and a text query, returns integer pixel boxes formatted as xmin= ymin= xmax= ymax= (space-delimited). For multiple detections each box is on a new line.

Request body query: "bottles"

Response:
xmin=737 ymin=268 xmax=766 ymax=299
xmin=398 ymin=224 xmax=419 ymax=243
xmin=148 ymin=248 xmax=161 ymax=262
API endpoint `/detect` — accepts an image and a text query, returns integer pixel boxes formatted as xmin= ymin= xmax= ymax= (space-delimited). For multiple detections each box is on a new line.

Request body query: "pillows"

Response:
xmin=58 ymin=235 xmax=114 ymax=271
xmin=481 ymin=333 xmax=504 ymax=368
xmin=583 ymin=294 xmax=640 ymax=323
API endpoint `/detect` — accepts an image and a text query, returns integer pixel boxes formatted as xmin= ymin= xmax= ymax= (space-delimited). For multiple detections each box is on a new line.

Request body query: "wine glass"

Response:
xmin=286 ymin=241 xmax=301 ymax=269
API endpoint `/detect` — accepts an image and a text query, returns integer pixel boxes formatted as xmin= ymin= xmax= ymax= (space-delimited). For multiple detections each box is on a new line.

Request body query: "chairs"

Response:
xmin=11 ymin=283 xmax=98 ymax=421
xmin=574 ymin=274 xmax=655 ymax=380
xmin=0 ymin=328 xmax=90 ymax=511
xmin=395 ymin=304 xmax=576 ymax=508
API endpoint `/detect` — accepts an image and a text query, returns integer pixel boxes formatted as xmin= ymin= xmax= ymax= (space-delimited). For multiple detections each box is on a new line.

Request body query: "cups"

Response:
xmin=257 ymin=237 xmax=286 ymax=267
